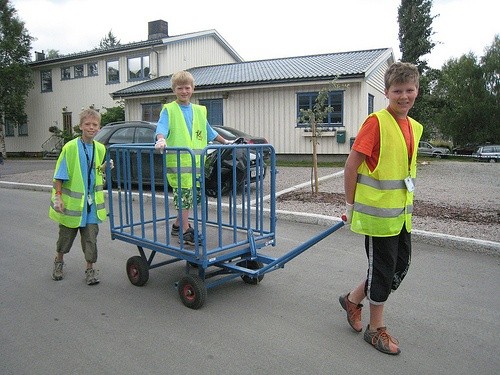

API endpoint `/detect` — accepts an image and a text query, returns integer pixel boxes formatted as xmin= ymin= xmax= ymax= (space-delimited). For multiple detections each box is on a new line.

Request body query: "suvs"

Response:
xmin=476 ymin=145 xmax=500 ymax=163
xmin=417 ymin=141 xmax=450 ymax=159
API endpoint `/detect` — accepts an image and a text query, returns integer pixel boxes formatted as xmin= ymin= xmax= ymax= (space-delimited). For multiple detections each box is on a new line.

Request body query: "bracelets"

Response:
xmin=55 ymin=191 xmax=62 ymax=195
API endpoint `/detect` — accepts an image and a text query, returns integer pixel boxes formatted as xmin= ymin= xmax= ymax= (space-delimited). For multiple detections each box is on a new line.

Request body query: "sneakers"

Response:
xmin=84 ymin=267 xmax=100 ymax=284
xmin=339 ymin=292 xmax=363 ymax=332
xmin=178 ymin=228 xmax=204 ymax=245
xmin=171 ymin=223 xmax=199 ymax=236
xmin=50 ymin=256 xmax=64 ymax=280
xmin=362 ymin=324 xmax=401 ymax=355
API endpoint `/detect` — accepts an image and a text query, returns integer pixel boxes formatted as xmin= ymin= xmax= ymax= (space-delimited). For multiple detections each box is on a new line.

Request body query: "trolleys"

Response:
xmin=104 ymin=142 xmax=347 ymax=311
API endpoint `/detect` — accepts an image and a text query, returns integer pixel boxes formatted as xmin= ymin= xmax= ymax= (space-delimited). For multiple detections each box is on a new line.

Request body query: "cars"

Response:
xmin=213 ymin=125 xmax=270 ymax=156
xmin=93 ymin=119 xmax=268 ymax=199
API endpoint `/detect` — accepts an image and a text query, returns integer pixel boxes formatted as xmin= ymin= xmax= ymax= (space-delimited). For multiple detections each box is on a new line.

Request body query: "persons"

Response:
xmin=48 ymin=109 xmax=114 ymax=286
xmin=155 ymin=71 xmax=235 ymax=246
xmin=338 ymin=62 xmax=423 ymax=355
